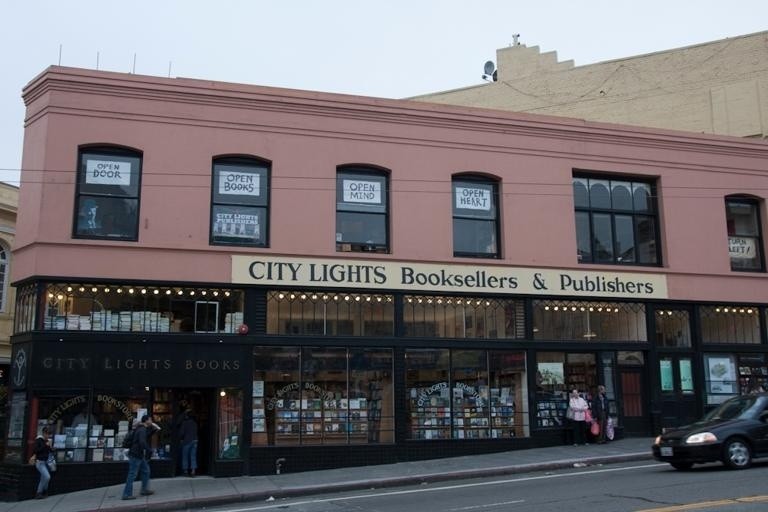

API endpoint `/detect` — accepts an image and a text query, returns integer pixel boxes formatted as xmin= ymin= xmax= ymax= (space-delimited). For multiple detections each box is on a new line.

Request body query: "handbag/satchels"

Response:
xmin=565 ymin=406 xmax=575 ymax=420
xmin=28 ymin=454 xmax=36 ymax=465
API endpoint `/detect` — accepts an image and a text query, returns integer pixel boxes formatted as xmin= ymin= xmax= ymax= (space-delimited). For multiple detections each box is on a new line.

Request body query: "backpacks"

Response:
xmin=122 ymin=425 xmax=145 ymax=448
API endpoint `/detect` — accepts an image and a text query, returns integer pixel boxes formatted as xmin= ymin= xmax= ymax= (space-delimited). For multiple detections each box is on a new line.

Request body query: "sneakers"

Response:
xmin=141 ymin=490 xmax=154 ymax=495
xmin=35 ymin=488 xmax=48 ymax=499
xmin=122 ymin=496 xmax=136 ymax=500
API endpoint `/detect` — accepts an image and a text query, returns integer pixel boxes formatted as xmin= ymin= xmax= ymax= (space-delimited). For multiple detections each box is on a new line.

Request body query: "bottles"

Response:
xmin=152 ymin=423 xmax=160 ymax=430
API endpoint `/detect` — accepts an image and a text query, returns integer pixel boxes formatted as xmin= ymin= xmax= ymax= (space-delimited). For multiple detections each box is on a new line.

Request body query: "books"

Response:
xmin=739 ymin=365 xmax=768 ymax=395
xmin=408 ymin=369 xmax=516 ymax=437
xmin=253 ymin=367 xmax=382 ymax=444
xmin=536 ymin=362 xmax=618 ymax=427
xmin=44 ymin=309 xmax=244 ymax=334
xmin=38 ymin=391 xmax=174 ymax=462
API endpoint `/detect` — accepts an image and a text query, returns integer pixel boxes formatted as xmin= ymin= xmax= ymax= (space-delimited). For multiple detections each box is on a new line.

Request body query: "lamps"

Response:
xmin=220 ymin=388 xmax=226 ymax=397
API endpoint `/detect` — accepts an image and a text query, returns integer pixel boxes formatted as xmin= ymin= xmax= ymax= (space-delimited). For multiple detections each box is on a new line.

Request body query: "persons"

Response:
xmin=179 ymin=408 xmax=198 ymax=475
xmin=122 ymin=415 xmax=161 ymax=500
xmin=568 ymin=389 xmax=590 ymax=446
xmin=592 ymin=384 xmax=609 ymax=444
xmin=33 ymin=426 xmax=52 ymax=500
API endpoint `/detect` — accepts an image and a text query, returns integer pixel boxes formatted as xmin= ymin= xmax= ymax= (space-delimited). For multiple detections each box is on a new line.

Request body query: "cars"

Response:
xmin=650 ymin=391 xmax=766 ymax=471
